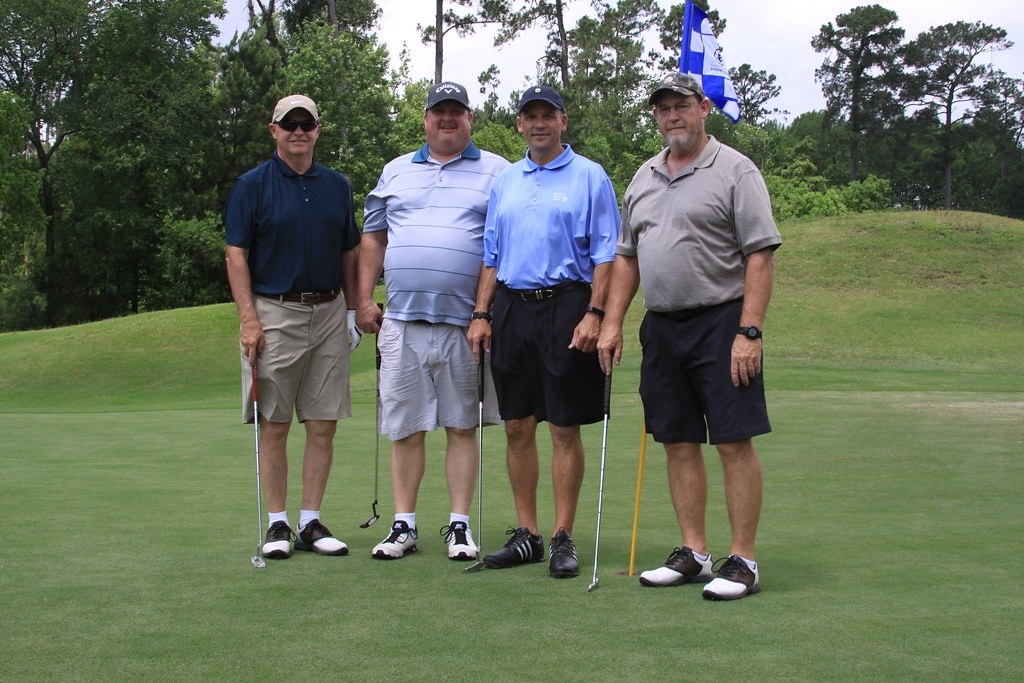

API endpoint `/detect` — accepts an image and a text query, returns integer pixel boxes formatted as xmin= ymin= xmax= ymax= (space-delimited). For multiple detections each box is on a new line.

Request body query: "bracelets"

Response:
xmin=584 ymin=306 xmax=604 ymax=320
xmin=471 ymin=312 xmax=491 ymax=324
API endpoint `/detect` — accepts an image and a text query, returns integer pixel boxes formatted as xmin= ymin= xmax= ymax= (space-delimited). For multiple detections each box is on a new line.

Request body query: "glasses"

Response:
xmin=273 ymin=119 xmax=318 ymax=132
xmin=654 ymin=100 xmax=700 ymax=114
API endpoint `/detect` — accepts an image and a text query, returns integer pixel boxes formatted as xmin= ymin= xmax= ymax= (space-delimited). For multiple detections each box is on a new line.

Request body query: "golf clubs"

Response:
xmin=251 ymin=355 xmax=266 ymax=569
xmin=360 ymin=303 xmax=381 ymax=530
xmin=462 ymin=342 xmax=485 ymax=572
xmin=588 ymin=350 xmax=614 ymax=590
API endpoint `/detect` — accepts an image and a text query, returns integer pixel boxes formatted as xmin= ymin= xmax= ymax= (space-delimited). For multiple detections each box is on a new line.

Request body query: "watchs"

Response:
xmin=737 ymin=327 xmax=762 ymax=339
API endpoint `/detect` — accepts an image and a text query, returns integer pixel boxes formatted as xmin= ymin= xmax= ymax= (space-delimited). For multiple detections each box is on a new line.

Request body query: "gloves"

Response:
xmin=346 ymin=308 xmax=360 ymax=352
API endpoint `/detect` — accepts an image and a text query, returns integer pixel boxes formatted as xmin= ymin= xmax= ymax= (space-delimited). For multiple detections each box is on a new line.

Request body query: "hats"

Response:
xmin=518 ymin=86 xmax=565 ymax=114
xmin=648 ymin=72 xmax=704 ymax=102
xmin=273 ymin=94 xmax=319 ymax=125
xmin=424 ymin=82 xmax=470 ymax=112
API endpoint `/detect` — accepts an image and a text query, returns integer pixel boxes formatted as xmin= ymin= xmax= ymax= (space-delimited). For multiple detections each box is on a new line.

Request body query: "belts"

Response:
xmin=252 ymin=290 xmax=339 ymax=306
xmin=501 ymin=282 xmax=579 ymax=302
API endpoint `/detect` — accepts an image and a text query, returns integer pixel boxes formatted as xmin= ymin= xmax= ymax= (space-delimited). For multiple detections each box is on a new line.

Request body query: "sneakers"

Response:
xmin=483 ymin=526 xmax=543 ymax=567
xmin=294 ymin=521 xmax=349 ymax=556
xmin=702 ymin=554 xmax=759 ymax=599
xmin=372 ymin=520 xmax=418 ymax=558
xmin=548 ymin=527 xmax=579 ymax=576
xmin=262 ymin=520 xmax=297 ymax=558
xmin=639 ymin=547 xmax=714 ymax=587
xmin=440 ymin=521 xmax=478 ymax=559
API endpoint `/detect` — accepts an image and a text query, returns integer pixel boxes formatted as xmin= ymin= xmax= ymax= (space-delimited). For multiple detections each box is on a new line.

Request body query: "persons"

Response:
xmin=352 ymin=81 xmax=512 ymax=559
xmin=226 ymin=94 xmax=361 ymax=556
xmin=596 ymin=72 xmax=782 ymax=599
xmin=465 ymin=88 xmax=621 ymax=575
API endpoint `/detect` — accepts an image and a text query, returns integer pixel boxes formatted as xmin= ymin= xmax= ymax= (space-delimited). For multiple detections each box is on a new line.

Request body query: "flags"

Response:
xmin=679 ymin=2 xmax=739 ymax=123
